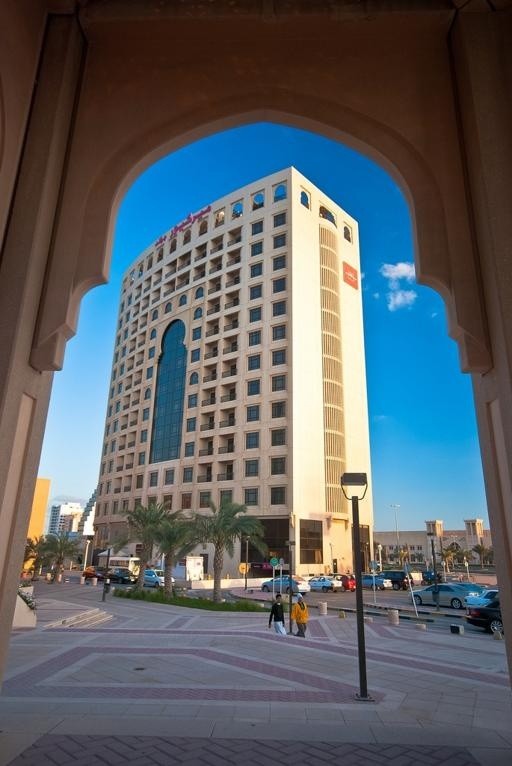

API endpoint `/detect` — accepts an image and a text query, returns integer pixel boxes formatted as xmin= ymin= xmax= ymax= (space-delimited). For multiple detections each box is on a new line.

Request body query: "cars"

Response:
xmin=82 ymin=566 xmax=175 ymax=588
xmin=261 ymin=570 xmax=414 ymax=597
xmin=407 ymin=582 xmax=504 ymax=635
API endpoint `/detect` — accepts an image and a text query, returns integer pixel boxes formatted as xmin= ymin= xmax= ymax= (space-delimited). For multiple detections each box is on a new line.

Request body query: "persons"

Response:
xmin=289 ymin=592 xmax=309 ymax=639
xmin=268 ymin=595 xmax=287 ymax=636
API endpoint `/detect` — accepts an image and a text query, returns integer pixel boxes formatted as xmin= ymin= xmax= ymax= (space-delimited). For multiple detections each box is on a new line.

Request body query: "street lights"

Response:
xmin=284 ymin=540 xmax=297 ymax=637
xmin=339 ymin=472 xmax=378 ymax=703
xmin=425 ymin=531 xmax=440 ymax=611
xmin=100 ymin=542 xmax=114 ymax=603
xmin=389 ymin=504 xmax=401 ymax=567
xmin=243 ymin=535 xmax=251 ymax=591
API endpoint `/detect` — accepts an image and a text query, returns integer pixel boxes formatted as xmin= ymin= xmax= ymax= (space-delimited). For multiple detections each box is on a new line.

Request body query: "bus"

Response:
xmin=109 ymin=556 xmax=141 ymax=579
